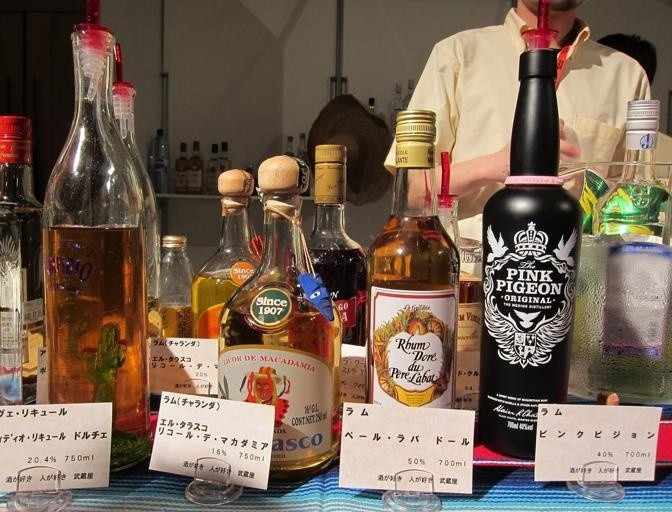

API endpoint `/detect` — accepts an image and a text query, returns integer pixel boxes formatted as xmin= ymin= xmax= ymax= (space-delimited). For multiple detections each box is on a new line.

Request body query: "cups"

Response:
xmin=560 ymin=161 xmax=672 ymax=404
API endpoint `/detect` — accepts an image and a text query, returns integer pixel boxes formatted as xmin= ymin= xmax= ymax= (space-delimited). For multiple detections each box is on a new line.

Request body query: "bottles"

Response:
xmin=586 ymin=101 xmax=671 ymax=403
xmin=366 ymin=75 xmax=485 ymax=483
xmin=49 ymin=21 xmax=161 ymax=470
xmin=0 ymin=113 xmax=45 ymax=412
xmin=147 ymin=128 xmax=367 ymax=482
xmin=478 ymin=42 xmax=584 ymax=461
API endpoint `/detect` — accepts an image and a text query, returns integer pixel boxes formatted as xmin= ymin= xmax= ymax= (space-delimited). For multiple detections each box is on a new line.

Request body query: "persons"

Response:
xmin=382 ymin=2 xmax=651 ymax=220
xmin=596 ymin=32 xmax=672 ymax=198
